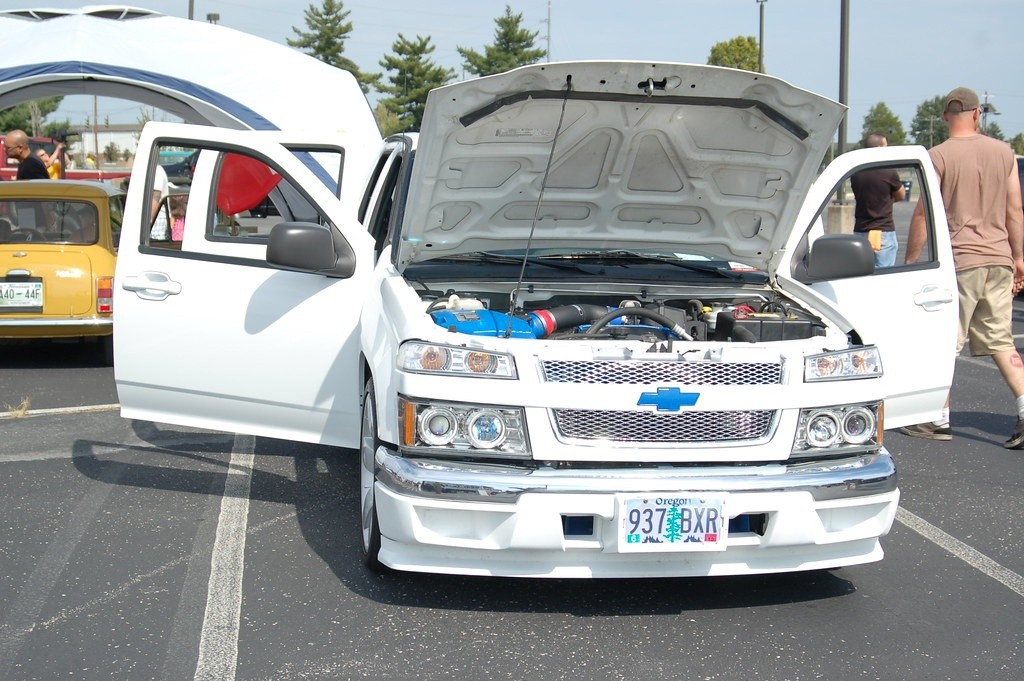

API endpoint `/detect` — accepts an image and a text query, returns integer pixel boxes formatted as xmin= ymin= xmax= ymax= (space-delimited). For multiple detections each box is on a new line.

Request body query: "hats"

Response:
xmin=944 ymin=87 xmax=979 ymax=112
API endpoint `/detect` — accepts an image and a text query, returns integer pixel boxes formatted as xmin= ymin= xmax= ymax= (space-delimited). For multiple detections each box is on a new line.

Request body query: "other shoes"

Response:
xmin=1004 ymin=414 xmax=1024 ymax=448
xmin=900 ymin=422 xmax=952 ymax=441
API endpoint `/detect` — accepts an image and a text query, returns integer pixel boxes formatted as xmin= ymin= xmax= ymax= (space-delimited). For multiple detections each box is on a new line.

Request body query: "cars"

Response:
xmin=113 ymin=58 xmax=962 ymax=579
xmin=0 ymin=130 xmax=283 ymax=364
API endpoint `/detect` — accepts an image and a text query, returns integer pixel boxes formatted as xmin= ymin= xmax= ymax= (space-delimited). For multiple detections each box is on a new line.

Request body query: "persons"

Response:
xmin=36 ymin=142 xmax=69 ymax=179
xmin=150 ymin=160 xmax=186 ymax=241
xmin=850 ymin=133 xmax=905 ymax=269
xmin=82 ymin=152 xmax=97 ymax=170
xmin=901 ymin=87 xmax=1024 ymax=447
xmin=4 ymin=131 xmax=59 ymax=234
